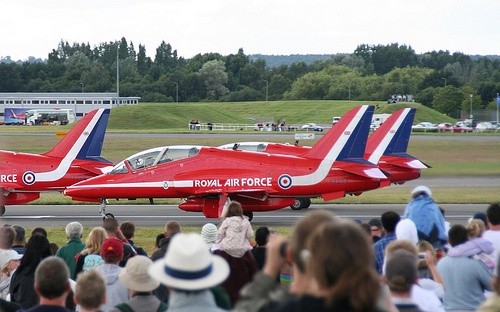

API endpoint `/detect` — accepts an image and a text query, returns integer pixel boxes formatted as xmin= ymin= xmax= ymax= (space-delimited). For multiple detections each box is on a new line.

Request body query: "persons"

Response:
xmin=0 ymin=185 xmax=500 ymax=312
xmin=135 ymin=158 xmax=143 ymax=169
xmin=190 ymin=119 xmax=287 ymax=131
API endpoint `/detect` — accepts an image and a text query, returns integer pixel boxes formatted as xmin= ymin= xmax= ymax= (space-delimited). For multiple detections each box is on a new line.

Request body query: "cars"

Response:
xmin=412 ymin=118 xmax=500 ymax=132
xmin=303 ymin=123 xmax=323 ymax=132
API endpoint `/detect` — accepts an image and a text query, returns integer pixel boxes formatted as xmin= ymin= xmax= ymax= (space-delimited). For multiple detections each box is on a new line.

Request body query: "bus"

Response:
xmin=22 ymin=109 xmax=76 ymax=126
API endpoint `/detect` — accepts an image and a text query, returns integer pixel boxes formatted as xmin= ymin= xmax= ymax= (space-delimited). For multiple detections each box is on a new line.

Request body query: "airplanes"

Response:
xmin=62 ymin=105 xmax=395 ymax=221
xmin=217 ymin=108 xmax=433 ymax=210
xmin=0 ymin=108 xmax=116 ymax=205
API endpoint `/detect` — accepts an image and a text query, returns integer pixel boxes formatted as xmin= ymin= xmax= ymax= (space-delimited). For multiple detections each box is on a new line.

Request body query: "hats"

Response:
xmin=0 ymin=250 xmax=23 ymax=268
xmin=201 ymin=224 xmax=218 ymax=242
xmin=82 ymin=254 xmax=102 ymax=271
xmin=118 ymin=255 xmax=160 ymax=292
xmin=101 ymin=238 xmax=122 ymax=256
xmin=148 ymin=232 xmax=230 ymax=291
xmin=395 ymin=219 xmax=417 ymax=246
xmin=412 ymin=186 xmax=431 ymax=197
xmin=385 ymin=249 xmax=416 ymax=286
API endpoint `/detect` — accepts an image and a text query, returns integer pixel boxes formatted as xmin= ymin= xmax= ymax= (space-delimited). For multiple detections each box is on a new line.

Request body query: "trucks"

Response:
xmin=370 ymin=114 xmax=392 ymax=131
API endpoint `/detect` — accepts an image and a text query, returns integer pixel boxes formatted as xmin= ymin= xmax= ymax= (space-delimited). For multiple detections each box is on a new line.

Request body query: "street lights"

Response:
xmin=173 ymin=82 xmax=179 ymax=103
xmin=264 ymin=80 xmax=268 ymax=102
xmin=441 ymin=78 xmax=446 ymax=88
xmin=469 ymin=94 xmax=473 ymax=128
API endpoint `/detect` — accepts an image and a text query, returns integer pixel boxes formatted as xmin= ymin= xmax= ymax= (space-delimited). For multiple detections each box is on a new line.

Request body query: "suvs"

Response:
xmin=333 ymin=116 xmax=341 ymax=125
